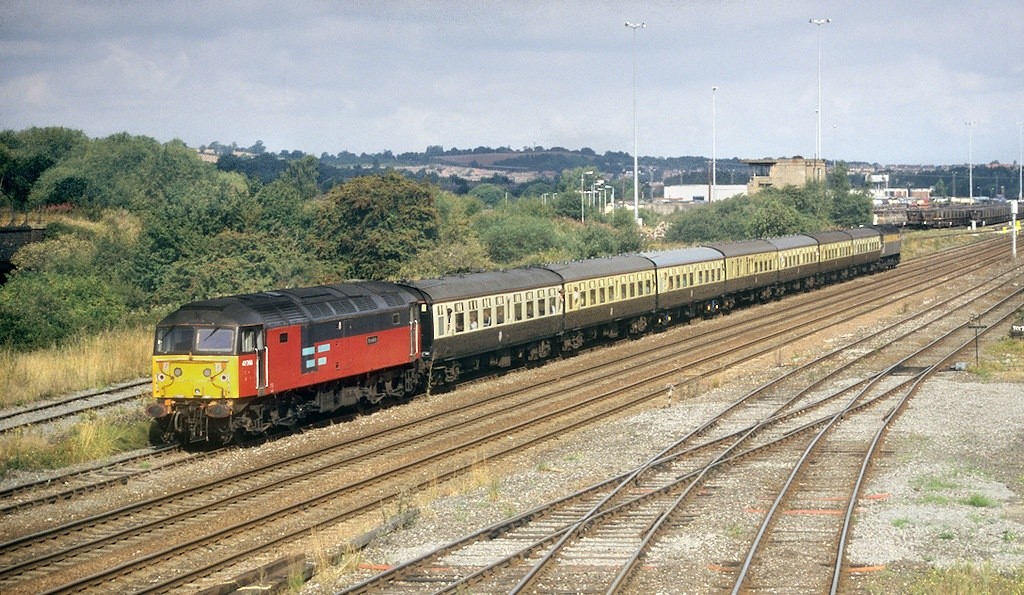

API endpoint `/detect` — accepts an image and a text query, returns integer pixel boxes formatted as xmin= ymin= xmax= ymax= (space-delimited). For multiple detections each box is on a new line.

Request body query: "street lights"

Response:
xmin=815 ymin=109 xmax=818 ymax=161
xmin=1016 ymin=121 xmax=1023 ymax=203
xmin=808 ymin=17 xmax=833 ymax=157
xmin=711 ymin=85 xmax=720 ymax=202
xmin=541 ymin=170 xmax=615 ymax=224
xmin=963 ymin=120 xmax=977 ymax=206
xmin=624 ymin=19 xmax=647 ymax=223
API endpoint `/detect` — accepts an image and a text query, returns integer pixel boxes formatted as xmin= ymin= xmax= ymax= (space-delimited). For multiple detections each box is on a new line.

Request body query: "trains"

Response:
xmin=147 ymin=223 xmax=906 ymax=454
